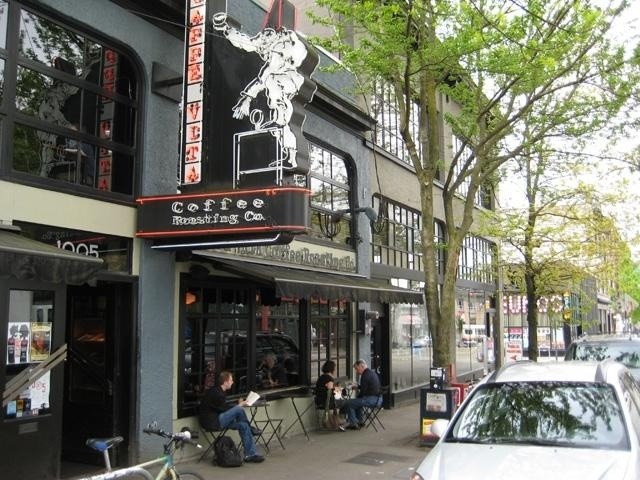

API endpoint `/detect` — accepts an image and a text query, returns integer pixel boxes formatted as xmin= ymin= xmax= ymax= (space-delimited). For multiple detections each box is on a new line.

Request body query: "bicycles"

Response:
xmin=71 ymin=421 xmax=208 ymax=480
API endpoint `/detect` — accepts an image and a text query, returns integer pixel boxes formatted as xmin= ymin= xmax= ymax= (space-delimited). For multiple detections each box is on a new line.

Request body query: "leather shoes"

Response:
xmin=250 ymin=426 xmax=262 ymax=436
xmin=244 ymin=454 xmax=265 ymax=463
xmin=345 ymin=423 xmax=359 ymax=430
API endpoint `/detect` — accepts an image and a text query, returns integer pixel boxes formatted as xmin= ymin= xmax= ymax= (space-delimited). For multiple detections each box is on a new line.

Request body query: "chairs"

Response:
xmin=196 ymin=385 xmax=386 ymax=464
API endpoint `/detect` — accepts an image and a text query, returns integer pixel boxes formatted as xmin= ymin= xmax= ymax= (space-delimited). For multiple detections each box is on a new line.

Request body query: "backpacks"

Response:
xmin=213 ymin=435 xmax=243 ymax=468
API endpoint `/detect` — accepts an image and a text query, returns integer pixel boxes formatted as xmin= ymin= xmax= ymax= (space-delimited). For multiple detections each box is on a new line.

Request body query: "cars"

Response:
xmin=409 ymin=360 xmax=640 ymax=480
xmin=391 ymin=333 xmax=493 ymax=361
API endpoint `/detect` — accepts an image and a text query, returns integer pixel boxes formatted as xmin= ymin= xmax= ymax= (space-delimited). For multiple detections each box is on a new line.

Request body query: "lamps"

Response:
xmin=332 ymin=206 xmax=378 ymax=223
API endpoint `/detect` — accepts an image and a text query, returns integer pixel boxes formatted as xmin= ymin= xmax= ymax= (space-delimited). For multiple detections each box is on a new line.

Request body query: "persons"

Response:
xmin=280 ymin=345 xmax=296 ymax=373
xmin=258 ymin=352 xmax=288 ymax=386
xmin=341 ymin=358 xmax=384 ymax=430
xmin=314 ymin=359 xmax=347 ymax=432
xmin=197 ymin=370 xmax=266 ymax=463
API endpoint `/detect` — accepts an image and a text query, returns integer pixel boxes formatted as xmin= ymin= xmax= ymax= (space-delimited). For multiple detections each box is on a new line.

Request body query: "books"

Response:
xmin=241 ymin=389 xmax=262 ymax=409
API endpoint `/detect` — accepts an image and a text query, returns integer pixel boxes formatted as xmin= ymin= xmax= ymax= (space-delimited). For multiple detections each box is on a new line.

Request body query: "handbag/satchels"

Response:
xmin=322 ymin=390 xmax=339 ymax=430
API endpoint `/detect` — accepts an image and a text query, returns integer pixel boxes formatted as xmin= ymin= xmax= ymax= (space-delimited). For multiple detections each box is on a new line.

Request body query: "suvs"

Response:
xmin=562 ymin=328 xmax=640 ymax=391
xmin=184 ymin=331 xmax=300 ymax=387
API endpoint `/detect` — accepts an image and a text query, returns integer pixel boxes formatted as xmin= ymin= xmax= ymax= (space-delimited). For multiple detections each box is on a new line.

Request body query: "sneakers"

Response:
xmin=339 ymin=424 xmax=345 ymax=431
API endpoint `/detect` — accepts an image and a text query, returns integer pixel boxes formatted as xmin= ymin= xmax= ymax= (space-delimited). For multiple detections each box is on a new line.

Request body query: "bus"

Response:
xmin=460 ymin=324 xmax=493 ymax=338
